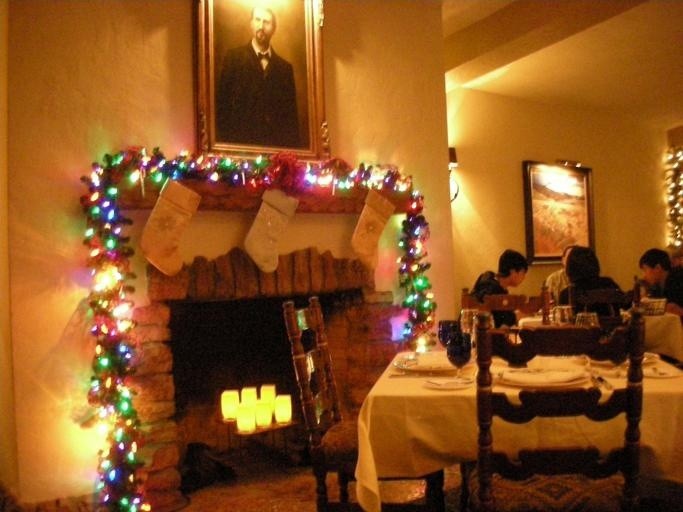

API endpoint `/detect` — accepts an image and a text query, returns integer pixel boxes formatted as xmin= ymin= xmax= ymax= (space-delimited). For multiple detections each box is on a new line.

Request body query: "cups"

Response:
xmin=574 ymin=312 xmax=602 ymax=345
xmin=552 ymin=305 xmax=574 ymax=329
xmin=437 ymin=320 xmax=462 ymax=348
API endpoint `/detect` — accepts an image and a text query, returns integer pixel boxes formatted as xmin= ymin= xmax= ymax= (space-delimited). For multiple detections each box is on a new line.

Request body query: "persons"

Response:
xmin=470 ymin=247 xmax=530 ymax=330
xmin=546 ymin=247 xmax=571 ymax=305
xmin=639 ymin=247 xmax=683 ymax=365
xmin=213 ymin=7 xmax=303 ymax=149
xmin=558 ymin=245 xmax=633 ymax=341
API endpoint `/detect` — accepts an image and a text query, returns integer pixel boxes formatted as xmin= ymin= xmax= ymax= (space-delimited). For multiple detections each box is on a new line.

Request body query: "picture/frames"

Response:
xmin=522 ymin=160 xmax=596 ymax=266
xmin=196 ymin=0 xmax=331 ymax=164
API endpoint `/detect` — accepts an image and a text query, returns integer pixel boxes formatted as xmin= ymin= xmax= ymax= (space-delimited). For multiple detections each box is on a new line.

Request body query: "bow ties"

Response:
xmin=258 ymin=52 xmax=271 ymax=61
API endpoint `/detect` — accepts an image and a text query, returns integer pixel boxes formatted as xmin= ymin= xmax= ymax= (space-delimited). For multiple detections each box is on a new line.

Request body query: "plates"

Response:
xmin=392 ymin=346 xmax=475 ymax=373
xmin=624 ymin=350 xmax=659 ymax=366
xmin=498 ymin=363 xmax=593 ymax=389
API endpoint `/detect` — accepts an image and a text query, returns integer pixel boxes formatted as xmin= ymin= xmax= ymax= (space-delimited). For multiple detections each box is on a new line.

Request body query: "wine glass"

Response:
xmin=447 ymin=332 xmax=472 ymax=382
